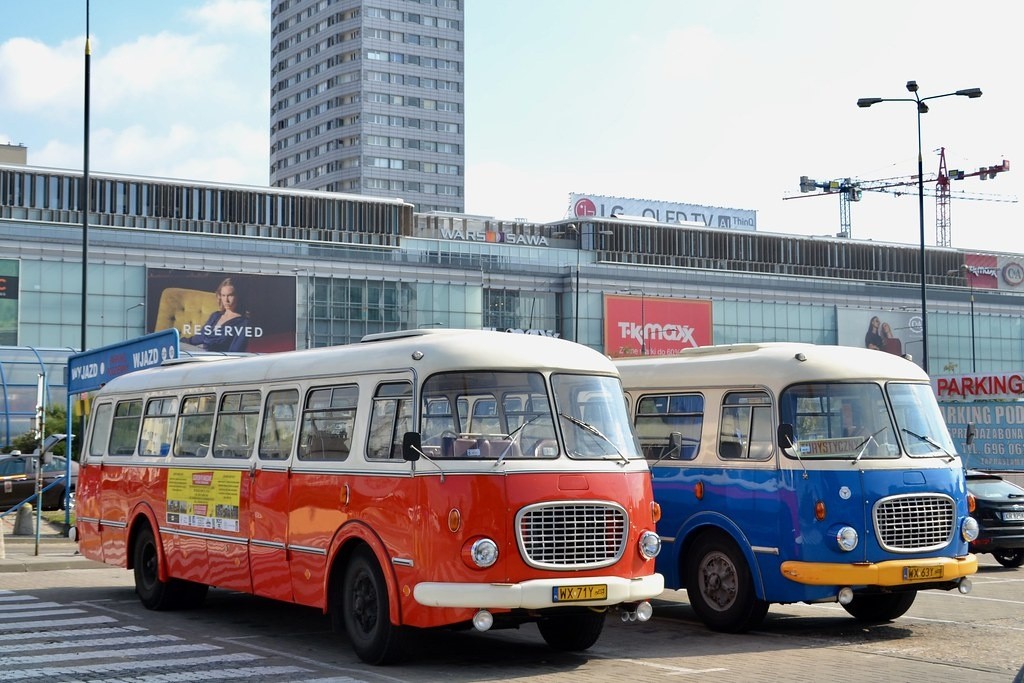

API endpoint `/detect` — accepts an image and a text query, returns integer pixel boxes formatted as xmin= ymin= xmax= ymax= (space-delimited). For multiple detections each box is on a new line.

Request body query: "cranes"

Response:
xmin=782 ymin=147 xmax=1009 ymax=249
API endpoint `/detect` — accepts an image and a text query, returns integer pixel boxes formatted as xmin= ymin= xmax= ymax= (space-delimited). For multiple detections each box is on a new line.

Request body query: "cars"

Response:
xmin=0 ymin=455 xmax=80 ymax=512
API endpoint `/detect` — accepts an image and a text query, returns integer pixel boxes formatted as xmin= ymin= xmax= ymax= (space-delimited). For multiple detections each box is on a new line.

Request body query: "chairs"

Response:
xmin=115 ymin=429 xmax=559 ymax=460
xmin=642 ymin=441 xmax=742 ymax=459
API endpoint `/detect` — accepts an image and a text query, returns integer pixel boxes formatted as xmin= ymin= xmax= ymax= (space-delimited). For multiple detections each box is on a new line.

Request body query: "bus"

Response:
xmin=421 ymin=342 xmax=978 ymax=635
xmin=72 ymin=326 xmax=665 ymax=667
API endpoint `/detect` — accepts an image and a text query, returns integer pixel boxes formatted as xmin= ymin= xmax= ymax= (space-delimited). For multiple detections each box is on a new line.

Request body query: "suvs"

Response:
xmin=962 ymin=469 xmax=1024 ymax=567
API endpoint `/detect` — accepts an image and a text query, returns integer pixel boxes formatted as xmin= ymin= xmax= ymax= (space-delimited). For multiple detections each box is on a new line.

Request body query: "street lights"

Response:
xmin=858 ymin=81 xmax=984 ymax=372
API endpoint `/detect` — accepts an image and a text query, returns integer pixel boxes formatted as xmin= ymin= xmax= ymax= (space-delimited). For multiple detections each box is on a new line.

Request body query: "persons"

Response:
xmin=180 ymin=278 xmax=253 ymax=352
xmin=865 ymin=316 xmax=902 ymax=356
